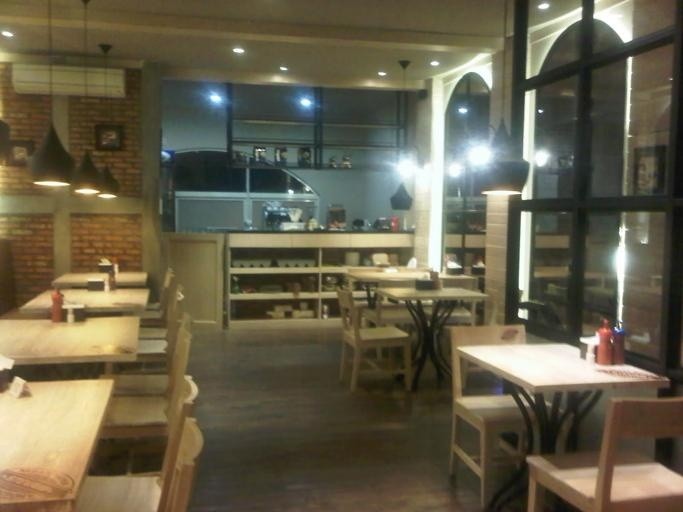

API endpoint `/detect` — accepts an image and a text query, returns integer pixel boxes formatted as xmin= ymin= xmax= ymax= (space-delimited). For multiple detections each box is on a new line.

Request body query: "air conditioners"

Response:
xmin=11 ymin=63 xmax=126 ymax=100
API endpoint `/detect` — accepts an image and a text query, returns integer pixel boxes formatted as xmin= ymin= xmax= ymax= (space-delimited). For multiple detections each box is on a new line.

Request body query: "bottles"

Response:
xmin=50 ymin=289 xmax=63 ymax=323
xmin=109 ymin=267 xmax=115 ymax=291
xmin=327 ymin=204 xmax=346 ymax=232
xmin=595 ymin=318 xmax=625 ymax=367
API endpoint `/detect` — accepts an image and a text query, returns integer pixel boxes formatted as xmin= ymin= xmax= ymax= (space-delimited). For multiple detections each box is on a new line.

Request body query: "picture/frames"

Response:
xmin=631 ymin=145 xmax=666 ymax=198
xmin=4 ymin=139 xmax=34 ymax=167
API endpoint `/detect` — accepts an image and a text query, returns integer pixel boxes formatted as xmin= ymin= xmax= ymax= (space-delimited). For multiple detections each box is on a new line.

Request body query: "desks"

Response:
xmin=0 ymin=272 xmax=150 ymax=512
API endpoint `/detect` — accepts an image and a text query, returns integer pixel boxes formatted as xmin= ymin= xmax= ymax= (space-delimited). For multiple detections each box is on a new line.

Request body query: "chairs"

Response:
xmin=67 ymin=267 xmax=205 ymax=511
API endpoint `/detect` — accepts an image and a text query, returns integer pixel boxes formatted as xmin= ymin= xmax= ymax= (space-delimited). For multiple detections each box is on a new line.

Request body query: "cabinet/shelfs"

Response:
xmin=224 ymin=231 xmax=415 ymax=331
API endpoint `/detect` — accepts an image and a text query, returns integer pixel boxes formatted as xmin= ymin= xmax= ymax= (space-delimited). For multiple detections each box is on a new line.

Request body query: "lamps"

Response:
xmin=474 ymin=0 xmax=529 ymax=197
xmin=29 ymin=0 xmax=122 ymax=201
xmin=390 ymin=59 xmax=414 ymax=211
